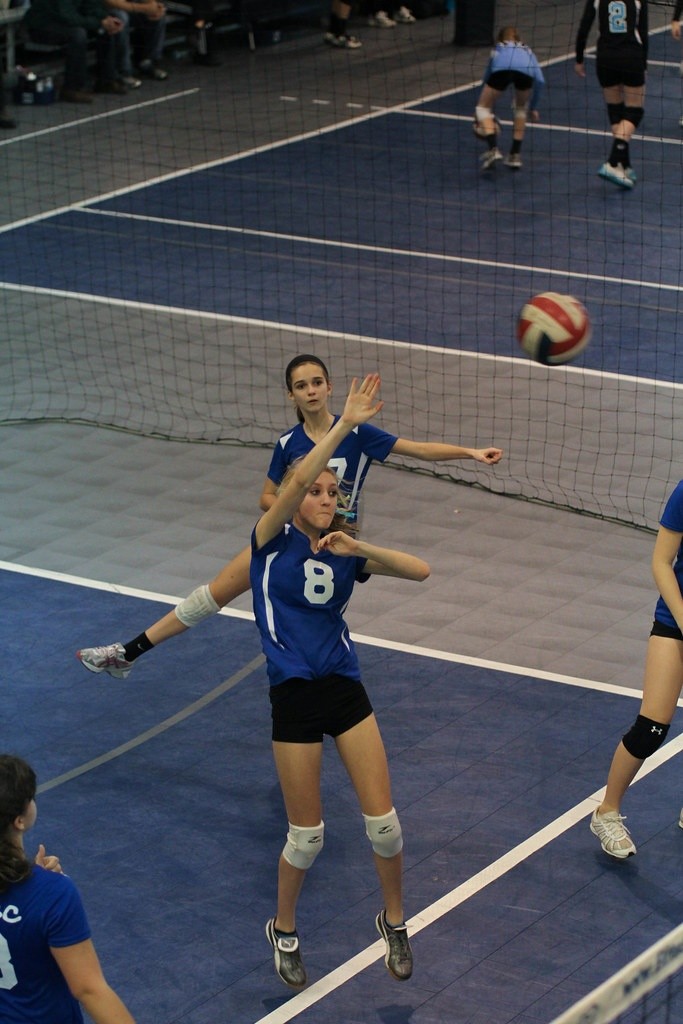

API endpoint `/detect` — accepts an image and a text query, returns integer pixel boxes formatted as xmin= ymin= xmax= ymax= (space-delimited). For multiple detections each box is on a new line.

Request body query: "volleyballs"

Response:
xmin=519 ymin=290 xmax=592 ymax=367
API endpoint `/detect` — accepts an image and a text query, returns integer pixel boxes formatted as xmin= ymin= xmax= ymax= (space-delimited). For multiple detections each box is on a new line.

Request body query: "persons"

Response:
xmin=0 ymin=754 xmax=135 ymax=1024
xmin=475 ymin=26 xmax=543 ymax=170
xmin=251 ymin=371 xmax=412 ymax=990
xmin=672 ymin=0 xmax=683 ymax=126
xmin=589 ymin=479 xmax=683 ymax=858
xmin=575 ymin=0 xmax=649 ymax=189
xmin=0 ymin=0 xmax=417 ymax=128
xmin=77 ymin=354 xmax=502 ymax=678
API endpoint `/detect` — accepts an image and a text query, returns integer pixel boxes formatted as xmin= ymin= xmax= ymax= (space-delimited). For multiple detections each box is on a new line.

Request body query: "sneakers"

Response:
xmin=502 ymin=152 xmax=523 ymax=168
xmin=375 ymin=908 xmax=413 ymax=981
xmin=75 ymin=642 xmax=136 ymax=680
xmin=597 ymin=162 xmax=637 ymax=189
xmin=590 ymin=805 xmax=637 ymax=859
xmin=264 ymin=913 xmax=307 ymax=988
xmin=481 ymin=146 xmax=504 ymax=170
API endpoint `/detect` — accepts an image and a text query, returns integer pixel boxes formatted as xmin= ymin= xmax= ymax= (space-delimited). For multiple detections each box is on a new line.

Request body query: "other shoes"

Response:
xmin=61 ymin=87 xmax=94 ymax=104
xmin=120 ymin=75 xmax=143 ymax=89
xmin=367 ymin=10 xmax=396 ymax=28
xmin=99 ymin=77 xmax=129 ymax=94
xmin=394 ymin=6 xmax=416 ymax=25
xmin=323 ymin=32 xmax=361 ymax=48
xmin=137 ymin=58 xmax=168 ymax=80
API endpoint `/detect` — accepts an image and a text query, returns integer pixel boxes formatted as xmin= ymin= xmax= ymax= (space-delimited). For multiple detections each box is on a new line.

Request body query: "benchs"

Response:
xmin=25 ymin=14 xmax=238 ymax=80
